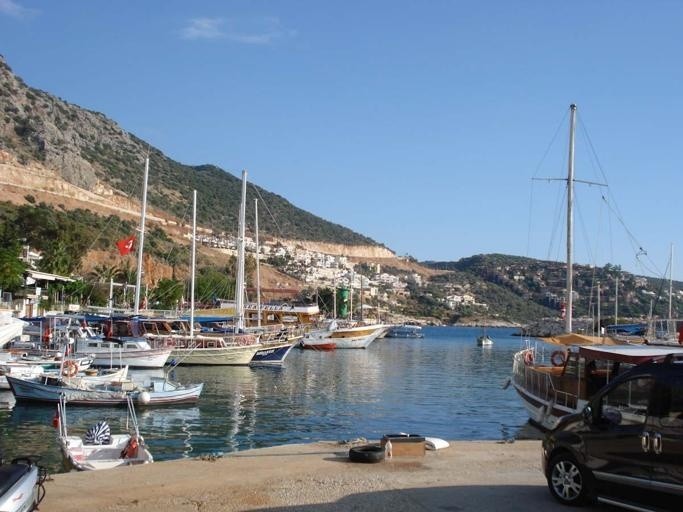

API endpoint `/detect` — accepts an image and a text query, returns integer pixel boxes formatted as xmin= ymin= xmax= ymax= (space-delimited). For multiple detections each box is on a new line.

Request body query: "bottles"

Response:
xmin=384 ymin=439 xmax=393 ymax=461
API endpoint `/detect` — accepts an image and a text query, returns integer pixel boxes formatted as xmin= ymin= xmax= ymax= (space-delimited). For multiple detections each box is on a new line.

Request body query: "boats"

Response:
xmin=477 ymin=335 xmax=495 ymax=346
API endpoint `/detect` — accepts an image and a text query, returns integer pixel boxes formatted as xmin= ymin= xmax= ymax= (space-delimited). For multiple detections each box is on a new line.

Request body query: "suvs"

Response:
xmin=534 ymin=354 xmax=682 ymax=512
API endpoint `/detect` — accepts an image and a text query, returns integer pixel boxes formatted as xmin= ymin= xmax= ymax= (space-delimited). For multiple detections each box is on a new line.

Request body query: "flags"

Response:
xmin=115 ymin=230 xmax=136 ymax=257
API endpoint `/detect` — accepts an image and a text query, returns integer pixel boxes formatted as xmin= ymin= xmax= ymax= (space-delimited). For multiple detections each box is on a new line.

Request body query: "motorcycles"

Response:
xmin=1 ymin=452 xmax=52 ymax=512
